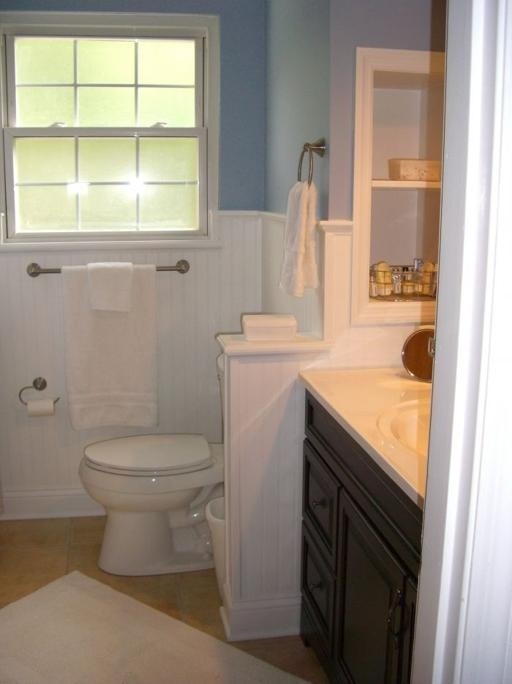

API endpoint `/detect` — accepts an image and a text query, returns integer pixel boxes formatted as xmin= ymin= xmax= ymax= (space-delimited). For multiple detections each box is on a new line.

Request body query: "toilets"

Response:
xmin=80 ymin=353 xmax=225 ymax=576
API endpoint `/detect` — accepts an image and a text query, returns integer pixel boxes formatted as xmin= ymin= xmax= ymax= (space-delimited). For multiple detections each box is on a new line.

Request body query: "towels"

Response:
xmin=277 ymin=182 xmax=319 ymax=299
xmin=60 ymin=261 xmax=158 ymax=431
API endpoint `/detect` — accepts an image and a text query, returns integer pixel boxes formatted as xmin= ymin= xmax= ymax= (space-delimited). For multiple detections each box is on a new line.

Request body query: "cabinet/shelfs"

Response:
xmin=298 ymin=391 xmax=424 ymax=683
xmin=348 ymin=45 xmax=446 ymax=328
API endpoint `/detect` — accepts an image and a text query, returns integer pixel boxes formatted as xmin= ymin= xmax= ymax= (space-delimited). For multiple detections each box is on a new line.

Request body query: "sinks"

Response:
xmin=377 ymin=403 xmax=429 ymax=458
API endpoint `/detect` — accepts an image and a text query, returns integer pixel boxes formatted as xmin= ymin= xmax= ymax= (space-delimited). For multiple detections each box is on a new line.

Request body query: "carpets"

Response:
xmin=0 ymin=571 xmax=312 ymax=684
